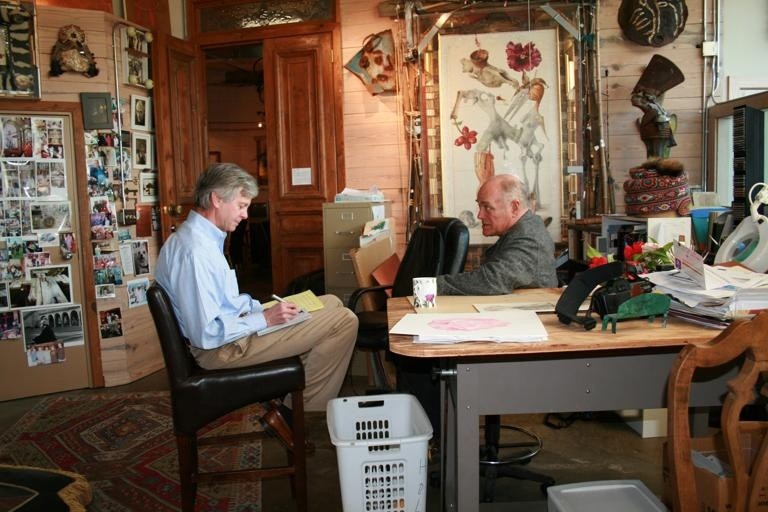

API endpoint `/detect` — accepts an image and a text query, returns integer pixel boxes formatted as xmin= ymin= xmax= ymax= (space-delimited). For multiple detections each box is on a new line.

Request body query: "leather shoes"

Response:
xmin=258 ymin=398 xmax=283 ymax=413
xmin=258 ymin=408 xmax=316 ymax=460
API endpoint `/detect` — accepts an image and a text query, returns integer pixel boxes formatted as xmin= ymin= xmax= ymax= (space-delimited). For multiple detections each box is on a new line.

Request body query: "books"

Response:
xmin=257 ymin=309 xmax=312 ymax=337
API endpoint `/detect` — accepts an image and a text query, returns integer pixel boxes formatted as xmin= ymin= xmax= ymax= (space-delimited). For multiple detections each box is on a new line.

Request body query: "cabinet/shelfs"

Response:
xmin=321 ymin=200 xmax=393 ymax=381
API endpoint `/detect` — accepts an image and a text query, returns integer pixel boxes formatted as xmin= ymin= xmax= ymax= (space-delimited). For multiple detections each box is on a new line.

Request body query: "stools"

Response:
xmin=425 ymin=408 xmax=555 ymax=507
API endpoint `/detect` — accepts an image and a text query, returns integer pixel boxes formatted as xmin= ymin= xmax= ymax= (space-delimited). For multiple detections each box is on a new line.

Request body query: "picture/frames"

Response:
xmin=130 ymin=93 xmax=148 ymax=131
xmin=139 ymin=173 xmax=159 ymax=205
xmin=132 ymin=133 xmax=152 ymax=170
xmin=79 ymin=91 xmax=114 ymax=130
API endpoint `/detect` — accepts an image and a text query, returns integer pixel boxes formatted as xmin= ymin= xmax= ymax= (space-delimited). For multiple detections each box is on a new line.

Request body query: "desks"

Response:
xmin=387 ymin=290 xmax=759 ymax=512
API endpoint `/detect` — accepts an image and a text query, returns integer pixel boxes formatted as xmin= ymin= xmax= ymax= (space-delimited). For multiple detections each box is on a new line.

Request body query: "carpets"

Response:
xmin=1 ymin=393 xmax=268 ymax=512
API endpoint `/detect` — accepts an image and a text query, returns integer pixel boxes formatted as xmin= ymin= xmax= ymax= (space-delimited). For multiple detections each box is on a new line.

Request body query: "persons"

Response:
xmin=26 ymin=342 xmax=66 ymax=367
xmin=104 ymin=312 xmax=112 ymax=323
xmin=90 ymin=160 xmax=109 ymax=183
xmin=135 ymin=101 xmax=145 ymax=124
xmin=397 ymin=175 xmax=557 ymax=463
xmin=137 ymin=143 xmax=146 ymax=164
xmin=154 ymin=164 xmax=358 ymax=458
xmin=32 ymin=314 xmax=59 ymax=344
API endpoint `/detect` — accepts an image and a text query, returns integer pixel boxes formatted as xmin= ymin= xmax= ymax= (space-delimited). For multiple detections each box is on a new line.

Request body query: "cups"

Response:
xmin=413 ymin=277 xmax=437 ymax=311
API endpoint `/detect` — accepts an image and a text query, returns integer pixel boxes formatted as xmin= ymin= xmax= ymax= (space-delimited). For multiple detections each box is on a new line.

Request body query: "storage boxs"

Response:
xmin=665 ymin=427 xmax=768 ymax=512
xmin=327 ymin=395 xmax=435 ymax=512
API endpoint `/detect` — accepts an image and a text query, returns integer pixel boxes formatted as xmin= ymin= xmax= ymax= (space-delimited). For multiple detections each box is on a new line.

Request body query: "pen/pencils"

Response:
xmin=272 ymin=294 xmax=303 ymax=313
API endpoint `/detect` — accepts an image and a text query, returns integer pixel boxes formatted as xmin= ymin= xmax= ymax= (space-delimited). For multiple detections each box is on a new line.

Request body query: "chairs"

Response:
xmin=346 ymin=218 xmax=468 ymax=430
xmin=146 ymin=287 xmax=307 ymax=510
xmin=664 ymin=314 xmax=768 ymax=512
